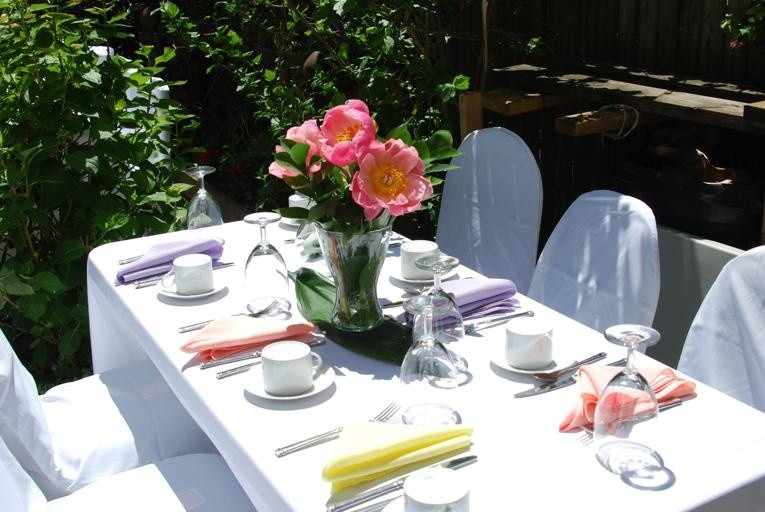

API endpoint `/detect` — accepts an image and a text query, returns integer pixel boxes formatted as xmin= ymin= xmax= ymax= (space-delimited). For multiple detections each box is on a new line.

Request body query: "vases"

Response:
xmin=314 ymin=223 xmax=392 ymax=334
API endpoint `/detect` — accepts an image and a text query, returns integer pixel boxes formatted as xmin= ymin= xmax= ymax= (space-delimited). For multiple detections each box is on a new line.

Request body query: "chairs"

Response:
xmin=524 ymin=187 xmax=664 ymax=358
xmin=0 ymin=433 xmax=258 ymax=512
xmin=0 ymin=326 xmax=212 ymax=495
xmin=676 ymin=240 xmax=764 ymax=414
xmin=435 ymin=127 xmax=547 ymax=296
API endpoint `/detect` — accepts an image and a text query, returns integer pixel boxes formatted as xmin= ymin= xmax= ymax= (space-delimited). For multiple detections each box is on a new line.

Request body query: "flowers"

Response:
xmin=268 ymin=97 xmax=434 ymax=327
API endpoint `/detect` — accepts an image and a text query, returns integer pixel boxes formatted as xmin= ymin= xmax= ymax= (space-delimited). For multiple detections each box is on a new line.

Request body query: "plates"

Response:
xmin=491 ymin=352 xmax=576 ymax=374
xmin=243 ymin=363 xmax=335 ymax=403
xmin=385 ymin=272 xmax=457 ymax=285
xmin=159 ymin=284 xmax=225 ymax=300
xmin=282 ymin=218 xmax=300 ymax=227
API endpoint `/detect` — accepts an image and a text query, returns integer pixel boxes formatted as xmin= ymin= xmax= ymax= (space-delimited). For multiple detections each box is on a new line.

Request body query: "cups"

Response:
xmin=259 ymin=341 xmax=321 ymax=395
xmin=402 ymin=238 xmax=440 ymax=277
xmin=289 ymin=194 xmax=310 ymax=208
xmin=162 ymin=253 xmax=213 ymax=294
xmin=507 ymin=316 xmax=552 ymax=367
xmin=403 ymin=467 xmax=470 ymax=512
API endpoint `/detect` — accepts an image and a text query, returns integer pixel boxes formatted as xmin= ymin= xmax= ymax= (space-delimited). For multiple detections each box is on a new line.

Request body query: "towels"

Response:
xmin=179 ymin=315 xmax=317 ymax=362
xmin=405 ymin=277 xmax=519 ymax=329
xmin=115 ymin=237 xmax=225 ymax=286
xmin=322 ymin=419 xmax=474 ymax=498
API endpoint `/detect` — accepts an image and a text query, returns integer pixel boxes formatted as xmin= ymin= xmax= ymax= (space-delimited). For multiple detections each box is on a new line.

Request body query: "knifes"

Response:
xmin=515 ymin=357 xmax=628 ymax=398
xmin=325 ymin=455 xmax=477 ymax=511
xmin=532 ymin=352 xmax=607 ymax=381
xmin=201 ymin=340 xmax=327 ymax=371
xmin=130 ymin=262 xmax=235 ymax=285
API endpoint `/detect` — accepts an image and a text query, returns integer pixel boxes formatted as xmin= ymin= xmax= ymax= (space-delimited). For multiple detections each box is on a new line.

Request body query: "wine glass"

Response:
xmin=180 ymin=166 xmax=225 ymax=233
xmin=594 ymin=323 xmax=663 ymax=480
xmin=401 ymin=253 xmax=464 ymax=426
xmin=243 ymin=211 xmax=290 ymax=315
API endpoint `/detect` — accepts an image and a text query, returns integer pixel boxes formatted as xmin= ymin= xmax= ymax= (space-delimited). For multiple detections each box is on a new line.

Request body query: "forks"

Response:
xmin=578 ymin=400 xmax=681 ymax=437
xmin=576 ymin=434 xmax=593 ymax=445
xmin=274 ymin=401 xmax=399 ymax=458
xmin=179 ymin=300 xmax=281 ymax=333
